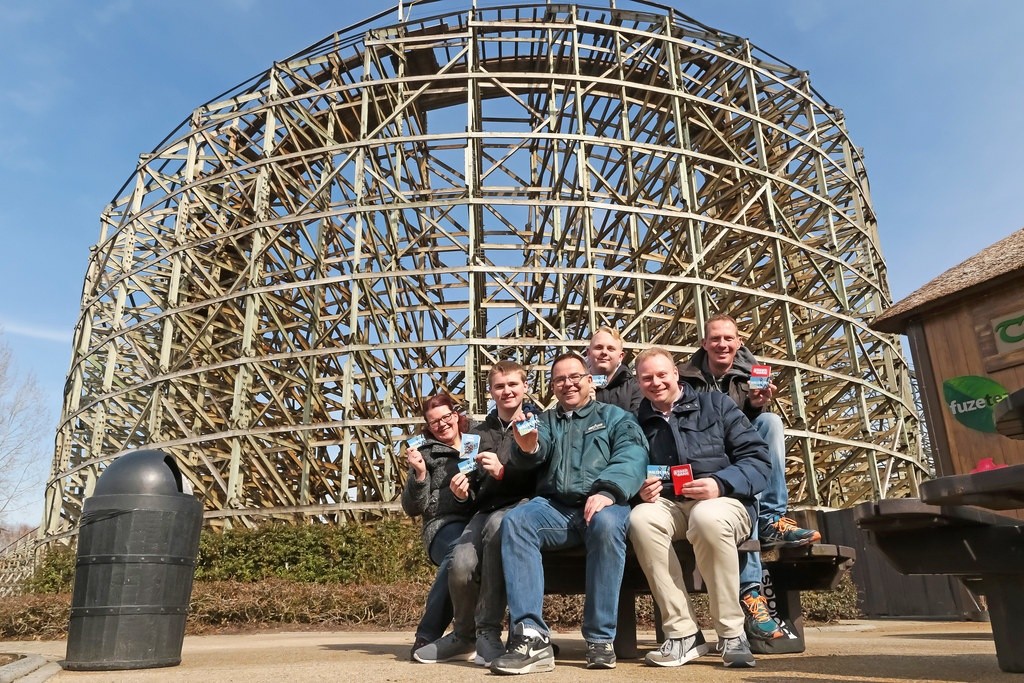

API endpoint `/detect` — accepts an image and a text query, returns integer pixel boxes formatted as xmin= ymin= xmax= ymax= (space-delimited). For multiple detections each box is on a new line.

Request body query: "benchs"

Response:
xmin=852 ymin=461 xmax=1024 ymax=674
xmin=533 ymin=541 xmax=857 ymax=659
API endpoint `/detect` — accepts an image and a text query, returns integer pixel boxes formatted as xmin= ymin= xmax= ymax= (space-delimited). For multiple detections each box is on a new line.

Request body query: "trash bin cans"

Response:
xmin=62 ymin=449 xmax=204 ymax=672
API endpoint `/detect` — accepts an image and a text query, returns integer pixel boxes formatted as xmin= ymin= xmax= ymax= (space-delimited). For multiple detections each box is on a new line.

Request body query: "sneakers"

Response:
xmin=489 ymin=623 xmax=555 ymax=675
xmin=413 ymin=630 xmax=476 ymax=664
xmin=758 ymin=516 xmax=821 ymax=547
xmin=474 ymin=627 xmax=506 ymax=667
xmin=715 ymin=636 xmax=756 ymax=668
xmin=736 ymin=588 xmax=784 ymax=640
xmin=584 ymin=639 xmax=617 ymax=668
xmin=644 ymin=629 xmax=709 ymax=667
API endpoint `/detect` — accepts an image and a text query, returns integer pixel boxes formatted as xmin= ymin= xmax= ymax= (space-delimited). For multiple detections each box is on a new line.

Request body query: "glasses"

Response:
xmin=428 ymin=411 xmax=452 ymax=429
xmin=551 ymin=373 xmax=589 ymax=387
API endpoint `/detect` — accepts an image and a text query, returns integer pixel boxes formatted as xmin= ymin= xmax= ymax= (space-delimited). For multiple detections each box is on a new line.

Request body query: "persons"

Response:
xmin=628 ymin=347 xmax=771 ymax=667
xmin=414 ymin=360 xmax=544 ymax=666
xmin=402 ymin=394 xmax=559 ymax=661
xmin=542 ymin=326 xmax=645 ymax=420
xmin=491 ymin=354 xmax=650 ymax=675
xmin=676 ymin=314 xmax=822 ymax=640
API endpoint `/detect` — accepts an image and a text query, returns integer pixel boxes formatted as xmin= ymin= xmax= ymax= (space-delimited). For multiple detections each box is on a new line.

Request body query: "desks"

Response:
xmin=992 ymin=386 xmax=1023 ymax=437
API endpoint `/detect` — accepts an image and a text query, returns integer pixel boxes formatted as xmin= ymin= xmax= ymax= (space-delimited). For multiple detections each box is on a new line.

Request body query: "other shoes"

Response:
xmin=411 ymin=638 xmax=430 ymax=661
